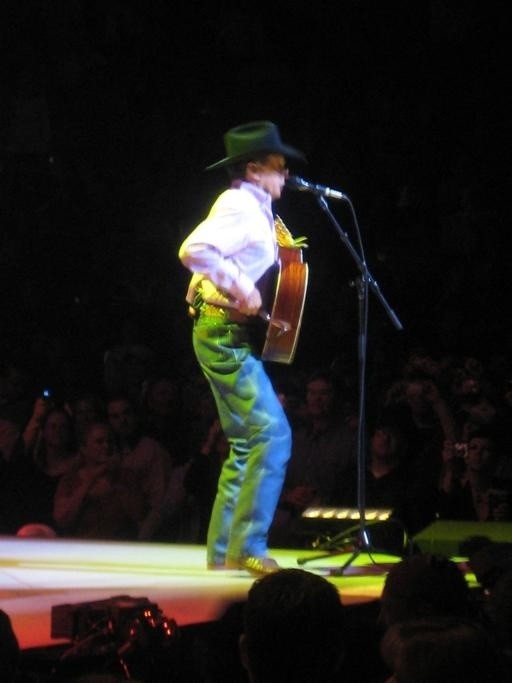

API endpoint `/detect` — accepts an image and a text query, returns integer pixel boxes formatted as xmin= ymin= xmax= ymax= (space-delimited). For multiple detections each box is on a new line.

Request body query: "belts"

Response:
xmin=199 ymin=302 xmax=246 ymax=322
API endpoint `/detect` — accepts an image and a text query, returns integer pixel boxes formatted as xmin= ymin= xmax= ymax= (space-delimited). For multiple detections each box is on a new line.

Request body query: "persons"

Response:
xmin=211 ymin=535 xmax=512 ymax=683
xmin=0 ymin=337 xmax=512 ymax=548
xmin=2 ymin=612 xmax=131 ymax=683
xmin=175 ymin=118 xmax=311 ymax=581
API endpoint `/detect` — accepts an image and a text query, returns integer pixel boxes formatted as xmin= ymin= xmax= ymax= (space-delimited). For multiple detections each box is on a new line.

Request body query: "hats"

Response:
xmin=204 ymin=120 xmax=301 ymax=169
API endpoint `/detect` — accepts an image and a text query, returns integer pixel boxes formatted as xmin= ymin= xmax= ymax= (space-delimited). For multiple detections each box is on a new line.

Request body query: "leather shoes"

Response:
xmin=224 ymin=555 xmax=280 ymax=578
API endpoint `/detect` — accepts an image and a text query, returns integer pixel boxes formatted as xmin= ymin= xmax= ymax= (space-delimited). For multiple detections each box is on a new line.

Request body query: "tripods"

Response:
xmin=297 ymin=195 xmax=403 ymax=576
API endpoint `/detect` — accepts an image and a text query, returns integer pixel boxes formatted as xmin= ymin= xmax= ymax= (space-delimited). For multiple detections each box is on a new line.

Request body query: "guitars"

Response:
xmin=261 ymin=213 xmax=309 ymax=365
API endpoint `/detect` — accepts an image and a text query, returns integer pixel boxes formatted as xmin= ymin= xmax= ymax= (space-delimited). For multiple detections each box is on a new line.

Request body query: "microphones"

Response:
xmin=284 ymin=175 xmax=343 ymax=200
